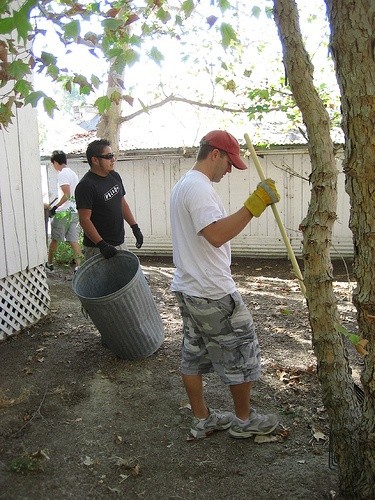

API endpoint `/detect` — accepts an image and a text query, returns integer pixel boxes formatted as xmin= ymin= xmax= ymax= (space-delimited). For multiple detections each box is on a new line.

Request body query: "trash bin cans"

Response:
xmin=71 ymin=250 xmax=165 ymax=360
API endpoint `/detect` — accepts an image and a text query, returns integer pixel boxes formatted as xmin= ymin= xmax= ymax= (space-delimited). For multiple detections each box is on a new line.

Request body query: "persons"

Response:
xmin=74 ymin=137 xmax=145 ymax=262
xmin=169 ymin=130 xmax=281 ymax=439
xmin=43 ymin=150 xmax=83 ymax=275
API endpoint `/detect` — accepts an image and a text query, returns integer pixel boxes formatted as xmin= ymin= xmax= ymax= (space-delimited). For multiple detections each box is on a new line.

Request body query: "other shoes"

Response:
xmin=69 ymin=259 xmax=80 ymax=271
xmin=45 ymin=263 xmax=55 ymax=275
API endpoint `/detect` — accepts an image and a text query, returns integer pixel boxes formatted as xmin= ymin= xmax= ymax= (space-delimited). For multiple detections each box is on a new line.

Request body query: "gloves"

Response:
xmin=244 ymin=178 xmax=281 ymax=217
xmin=130 ymin=224 xmax=144 ymax=249
xmin=96 ymin=240 xmax=118 ymax=259
xmin=49 ymin=206 xmax=57 ymax=218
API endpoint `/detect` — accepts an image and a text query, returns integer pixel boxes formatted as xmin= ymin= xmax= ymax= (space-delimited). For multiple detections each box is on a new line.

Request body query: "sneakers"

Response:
xmin=189 ymin=406 xmax=233 ymax=439
xmin=228 ymin=409 xmax=280 ymax=438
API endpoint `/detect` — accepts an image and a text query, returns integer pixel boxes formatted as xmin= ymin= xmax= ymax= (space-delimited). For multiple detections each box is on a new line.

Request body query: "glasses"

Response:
xmin=93 ymin=153 xmax=114 ymax=159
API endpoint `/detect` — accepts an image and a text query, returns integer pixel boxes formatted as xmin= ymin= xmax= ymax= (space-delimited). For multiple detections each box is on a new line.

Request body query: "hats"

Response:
xmin=198 ymin=129 xmax=248 ymax=171
